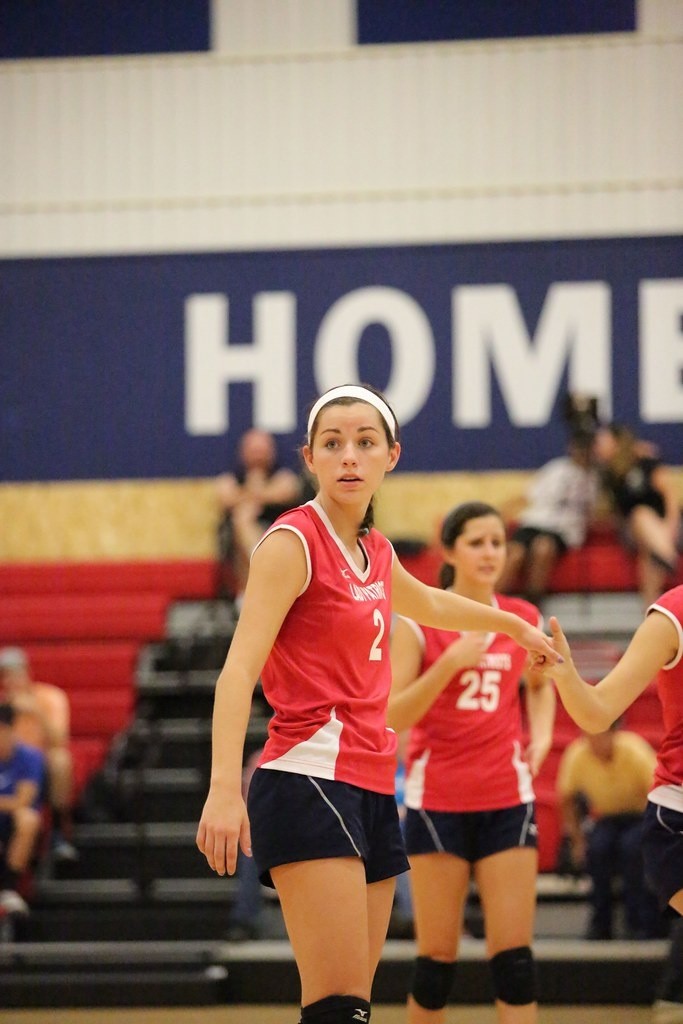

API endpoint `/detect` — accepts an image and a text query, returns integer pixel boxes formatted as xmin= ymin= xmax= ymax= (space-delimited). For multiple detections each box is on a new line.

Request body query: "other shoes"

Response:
xmin=0 ymin=891 xmax=29 ymax=916
xmin=55 ymin=842 xmax=76 ymax=859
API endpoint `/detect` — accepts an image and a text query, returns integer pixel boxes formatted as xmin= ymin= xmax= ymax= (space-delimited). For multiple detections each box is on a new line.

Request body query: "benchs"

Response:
xmin=0 ymin=539 xmax=683 ymax=1023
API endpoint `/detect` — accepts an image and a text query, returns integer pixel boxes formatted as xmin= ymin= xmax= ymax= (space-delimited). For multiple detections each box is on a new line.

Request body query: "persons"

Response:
xmin=528 ymin=585 xmax=683 ymax=1024
xmin=195 ymin=383 xmax=565 ymax=1024
xmin=495 ymin=424 xmax=677 ymax=618
xmin=0 ymin=646 xmax=75 ymax=916
xmin=387 ymin=501 xmax=555 ymax=1023
